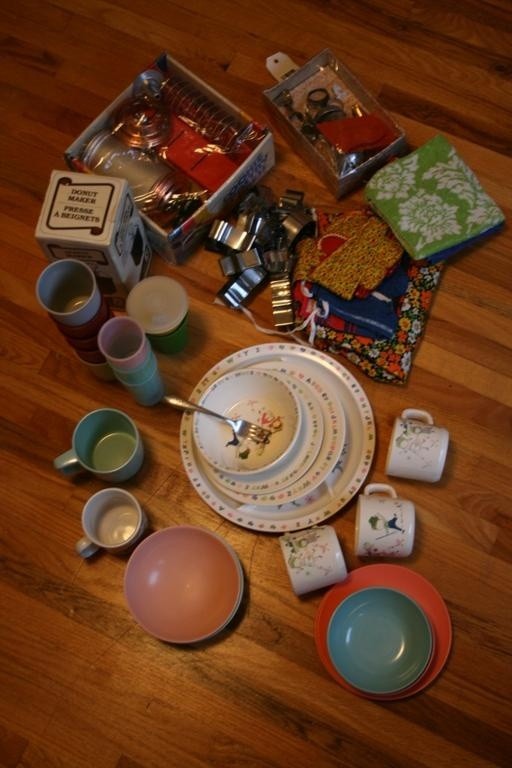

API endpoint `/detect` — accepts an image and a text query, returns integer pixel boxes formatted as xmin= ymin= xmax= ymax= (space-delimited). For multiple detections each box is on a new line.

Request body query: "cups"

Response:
xmin=354 ymin=483 xmax=415 ymax=559
xmin=52 ymin=407 xmax=145 ymax=483
xmin=125 ymin=275 xmax=192 ymax=356
xmin=98 ymin=315 xmax=167 ymax=407
xmin=35 ymin=258 xmax=120 ymax=382
xmin=280 ymin=525 xmax=348 ymax=597
xmin=75 ymin=486 xmax=148 ymax=560
xmin=384 ymin=408 xmax=451 ymax=484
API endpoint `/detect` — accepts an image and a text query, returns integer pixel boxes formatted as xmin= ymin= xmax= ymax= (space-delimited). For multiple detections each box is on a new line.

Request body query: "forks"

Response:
xmin=164 ymin=393 xmax=271 ymax=443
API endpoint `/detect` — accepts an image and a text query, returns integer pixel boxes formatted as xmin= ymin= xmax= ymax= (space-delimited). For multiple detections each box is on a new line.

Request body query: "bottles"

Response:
xmin=83 ymin=128 xmax=192 ymax=224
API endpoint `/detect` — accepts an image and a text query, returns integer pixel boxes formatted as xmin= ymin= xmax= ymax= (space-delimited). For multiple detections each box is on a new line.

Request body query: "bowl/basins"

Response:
xmin=191 ymin=368 xmax=303 ymax=479
xmin=327 ymin=586 xmax=437 ymax=697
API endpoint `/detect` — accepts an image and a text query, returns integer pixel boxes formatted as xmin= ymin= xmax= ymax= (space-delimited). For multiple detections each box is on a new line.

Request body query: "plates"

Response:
xmin=193 ymin=367 xmax=347 ymax=508
xmin=178 ymin=342 xmax=377 ymax=534
xmin=123 ymin=525 xmax=245 ymax=645
xmin=315 ymin=562 xmax=454 ymax=702
